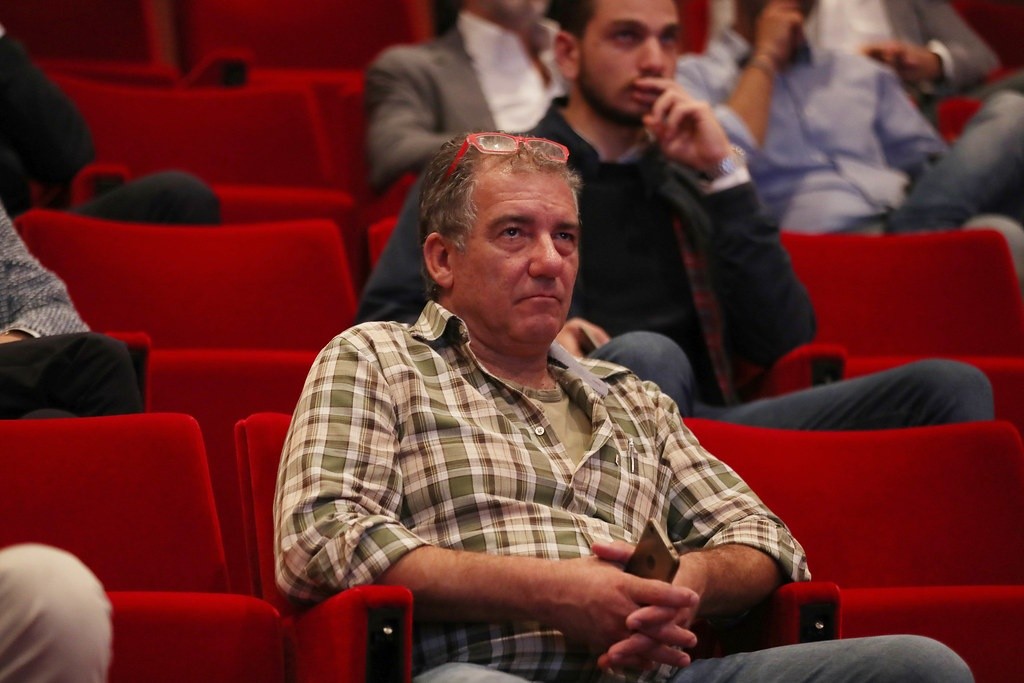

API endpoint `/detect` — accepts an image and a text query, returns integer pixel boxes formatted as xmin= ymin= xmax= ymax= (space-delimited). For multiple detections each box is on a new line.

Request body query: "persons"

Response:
xmin=0 ymin=543 xmax=111 ymax=683
xmin=0 ymin=198 xmax=144 ymax=420
xmin=0 ymin=24 xmax=219 ymax=225
xmin=351 ymin=0 xmax=1024 ymax=431
xmin=273 ymin=133 xmax=974 ymax=683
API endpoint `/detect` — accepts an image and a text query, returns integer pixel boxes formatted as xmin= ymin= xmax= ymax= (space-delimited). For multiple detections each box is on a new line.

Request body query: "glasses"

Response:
xmin=441 ymin=132 xmax=569 ymax=182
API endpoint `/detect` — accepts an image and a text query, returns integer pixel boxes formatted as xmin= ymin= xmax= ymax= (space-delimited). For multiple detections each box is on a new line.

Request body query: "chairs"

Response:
xmin=0 ymin=0 xmax=1024 ymax=683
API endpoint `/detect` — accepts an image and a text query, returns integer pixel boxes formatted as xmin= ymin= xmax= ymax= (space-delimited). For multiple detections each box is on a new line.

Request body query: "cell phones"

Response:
xmin=623 ymin=517 xmax=680 ymax=584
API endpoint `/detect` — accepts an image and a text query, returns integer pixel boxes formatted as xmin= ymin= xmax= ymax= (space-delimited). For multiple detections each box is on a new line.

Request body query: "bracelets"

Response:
xmin=706 ymin=143 xmax=749 ymax=181
xmin=733 ymin=47 xmax=786 ymax=82
